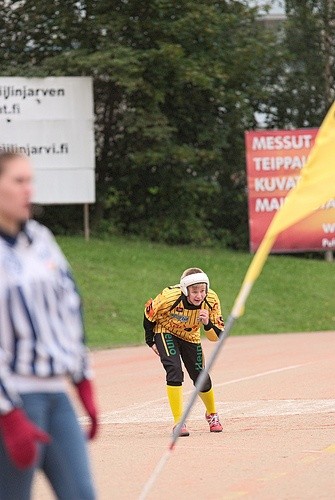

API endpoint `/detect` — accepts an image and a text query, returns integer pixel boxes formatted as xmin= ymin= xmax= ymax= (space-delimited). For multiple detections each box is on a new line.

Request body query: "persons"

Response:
xmin=0 ymin=149 xmax=102 ymax=500
xmin=144 ymin=265 xmax=226 ymax=439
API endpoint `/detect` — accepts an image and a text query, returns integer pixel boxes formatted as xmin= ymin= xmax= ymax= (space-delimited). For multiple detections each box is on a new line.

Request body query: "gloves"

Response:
xmin=76 ymin=379 xmax=98 ymax=439
xmin=0 ymin=410 xmax=51 ymax=469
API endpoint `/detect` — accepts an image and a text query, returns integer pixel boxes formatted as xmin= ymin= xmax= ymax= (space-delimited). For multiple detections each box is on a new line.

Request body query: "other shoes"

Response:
xmin=174 ymin=423 xmax=189 ymax=436
xmin=205 ymin=411 xmax=223 ymax=432
